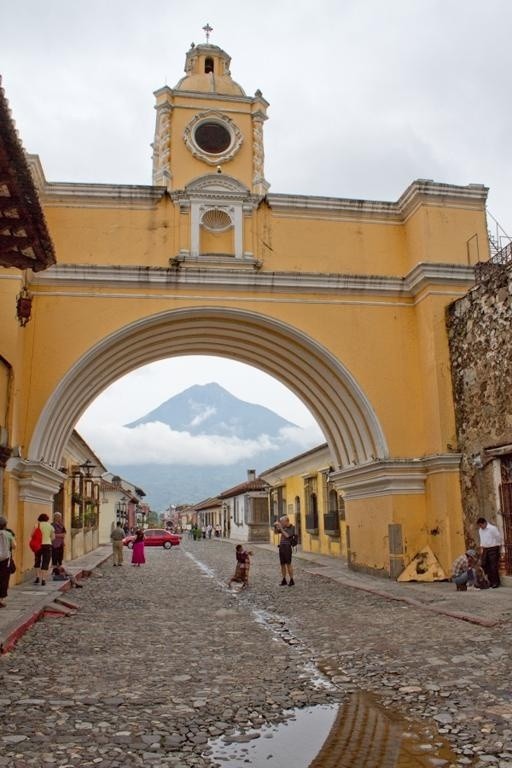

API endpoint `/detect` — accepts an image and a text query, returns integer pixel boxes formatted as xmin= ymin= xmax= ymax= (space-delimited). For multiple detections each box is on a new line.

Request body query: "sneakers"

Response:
xmin=33 ymin=576 xmax=40 ymax=584
xmin=279 ymin=577 xmax=288 ymax=586
xmin=456 ymin=581 xmax=502 ymax=591
xmin=287 ymin=578 xmax=296 ymax=586
xmin=41 ymin=579 xmax=46 ymax=585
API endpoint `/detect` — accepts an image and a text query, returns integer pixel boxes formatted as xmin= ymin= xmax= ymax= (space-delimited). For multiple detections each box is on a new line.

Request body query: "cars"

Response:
xmin=121 ymin=526 xmax=182 ymax=550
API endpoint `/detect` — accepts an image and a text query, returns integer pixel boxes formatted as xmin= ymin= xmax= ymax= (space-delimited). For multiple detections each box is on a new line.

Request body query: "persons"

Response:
xmin=227 ymin=545 xmax=255 ymax=586
xmin=0 ymin=516 xmax=17 ymax=607
xmin=272 ymin=515 xmax=296 ymax=586
xmin=450 ymin=549 xmax=489 ymax=587
xmin=111 ymin=521 xmax=146 ymax=567
xmin=28 ymin=514 xmax=56 ymax=585
xmin=191 ymin=524 xmax=221 ymax=540
xmin=52 ymin=510 xmax=66 ymax=572
xmin=477 ymin=517 xmax=503 ymax=587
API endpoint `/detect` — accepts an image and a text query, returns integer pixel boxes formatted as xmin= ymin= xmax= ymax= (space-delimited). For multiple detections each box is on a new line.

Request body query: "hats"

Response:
xmin=466 ymin=547 xmax=478 ymax=559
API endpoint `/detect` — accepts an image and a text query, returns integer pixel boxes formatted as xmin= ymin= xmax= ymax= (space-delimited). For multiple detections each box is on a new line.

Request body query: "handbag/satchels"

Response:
xmin=29 ymin=522 xmax=44 ymax=551
xmin=290 ymin=532 xmax=300 ymax=548
xmin=6 ymin=558 xmax=17 ymax=575
